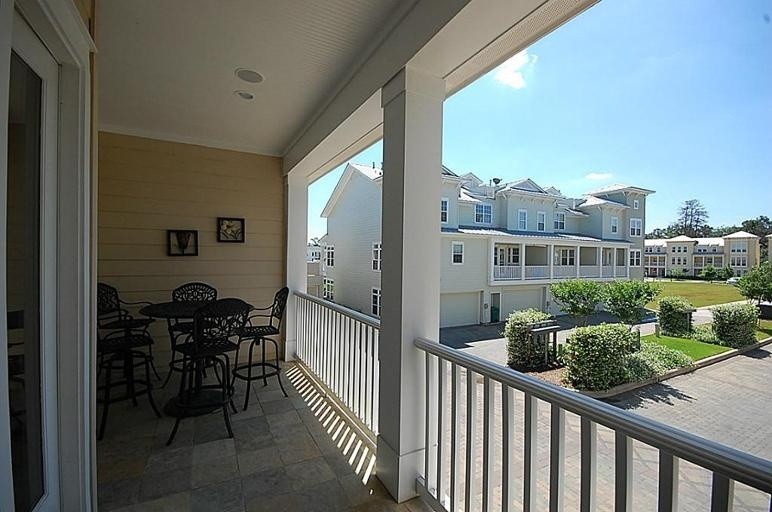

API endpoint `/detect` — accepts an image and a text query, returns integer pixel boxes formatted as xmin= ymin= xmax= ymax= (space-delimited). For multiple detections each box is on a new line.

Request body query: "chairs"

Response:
xmin=97 ymin=280 xmax=289 ymax=446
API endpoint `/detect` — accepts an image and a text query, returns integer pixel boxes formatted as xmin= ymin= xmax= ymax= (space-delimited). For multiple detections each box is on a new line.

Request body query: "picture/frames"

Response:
xmin=166 ymin=228 xmax=199 ymax=256
xmin=216 ymin=216 xmax=245 ymax=243
